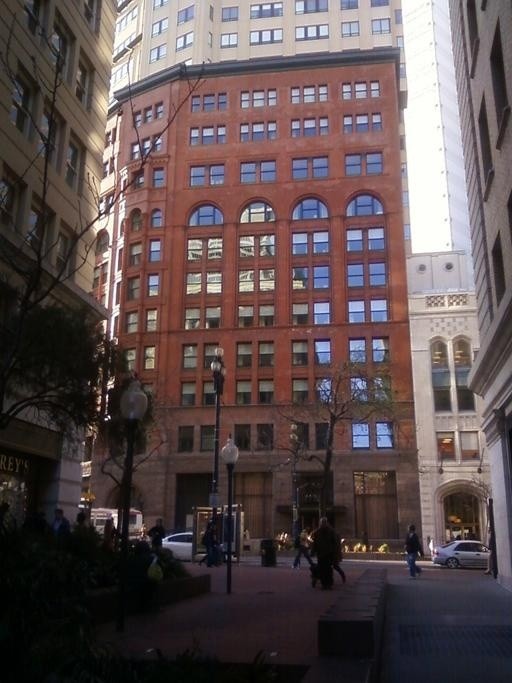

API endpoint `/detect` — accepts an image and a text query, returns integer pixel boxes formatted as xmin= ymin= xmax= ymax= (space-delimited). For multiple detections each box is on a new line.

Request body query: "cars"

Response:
xmin=431 ymin=540 xmax=489 ymax=569
xmin=161 ymin=533 xmax=237 ymax=562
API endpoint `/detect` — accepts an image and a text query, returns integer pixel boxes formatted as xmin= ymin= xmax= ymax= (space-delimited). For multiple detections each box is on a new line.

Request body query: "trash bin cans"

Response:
xmin=260 ymin=539 xmax=277 ymax=567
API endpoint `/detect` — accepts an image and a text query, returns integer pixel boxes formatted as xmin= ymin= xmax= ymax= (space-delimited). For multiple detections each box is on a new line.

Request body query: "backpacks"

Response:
xmin=294 ymin=537 xmax=300 ymax=548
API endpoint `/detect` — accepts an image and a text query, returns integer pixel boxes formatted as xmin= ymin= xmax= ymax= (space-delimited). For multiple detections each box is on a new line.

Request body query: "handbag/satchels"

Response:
xmin=201 ymin=535 xmax=207 ymax=545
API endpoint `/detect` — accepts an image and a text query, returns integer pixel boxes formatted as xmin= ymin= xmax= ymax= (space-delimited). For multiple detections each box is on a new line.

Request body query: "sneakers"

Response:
xmin=321 ymin=583 xmax=334 ymax=591
xmin=409 ymin=568 xmax=423 ymax=580
xmin=198 ymin=560 xmax=221 ymax=568
xmin=292 ymin=564 xmax=299 ymax=569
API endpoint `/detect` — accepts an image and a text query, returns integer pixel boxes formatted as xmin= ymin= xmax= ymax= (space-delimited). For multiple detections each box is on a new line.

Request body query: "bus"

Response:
xmin=90 ymin=508 xmax=150 ymax=545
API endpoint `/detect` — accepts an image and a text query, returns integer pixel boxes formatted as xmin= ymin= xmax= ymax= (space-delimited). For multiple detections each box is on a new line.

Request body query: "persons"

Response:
xmin=483 ymin=526 xmax=493 ymax=575
xmin=291 ymin=516 xmax=348 ymax=589
xmin=403 ymin=523 xmax=424 ymax=580
xmin=52 ymin=509 xmax=221 ymax=568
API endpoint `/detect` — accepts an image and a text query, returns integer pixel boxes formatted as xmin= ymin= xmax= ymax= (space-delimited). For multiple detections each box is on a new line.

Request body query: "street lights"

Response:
xmin=117 ymin=379 xmax=148 ymax=538
xmin=289 ymin=423 xmax=300 ymax=550
xmin=351 ymin=471 xmax=367 ymax=536
xmin=210 ymin=346 xmax=239 ymax=594
